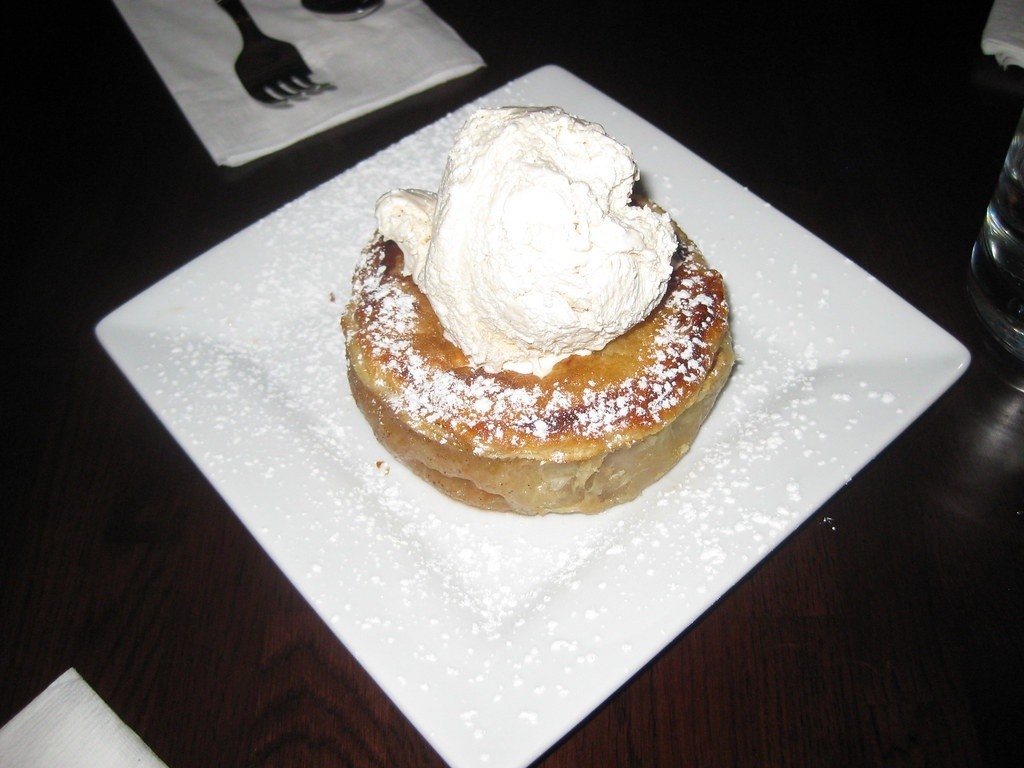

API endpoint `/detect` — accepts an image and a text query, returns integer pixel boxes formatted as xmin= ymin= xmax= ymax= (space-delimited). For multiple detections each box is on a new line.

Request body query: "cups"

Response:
xmin=299 ymin=0 xmax=385 ymax=21
xmin=967 ymin=117 xmax=1024 ymax=363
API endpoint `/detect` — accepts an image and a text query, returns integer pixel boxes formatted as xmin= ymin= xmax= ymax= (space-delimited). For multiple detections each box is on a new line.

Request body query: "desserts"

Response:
xmin=341 ymin=106 xmax=735 ymax=514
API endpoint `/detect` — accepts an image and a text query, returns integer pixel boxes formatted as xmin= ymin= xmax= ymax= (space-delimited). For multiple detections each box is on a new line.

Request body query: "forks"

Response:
xmin=215 ymin=0 xmax=331 ymax=103
